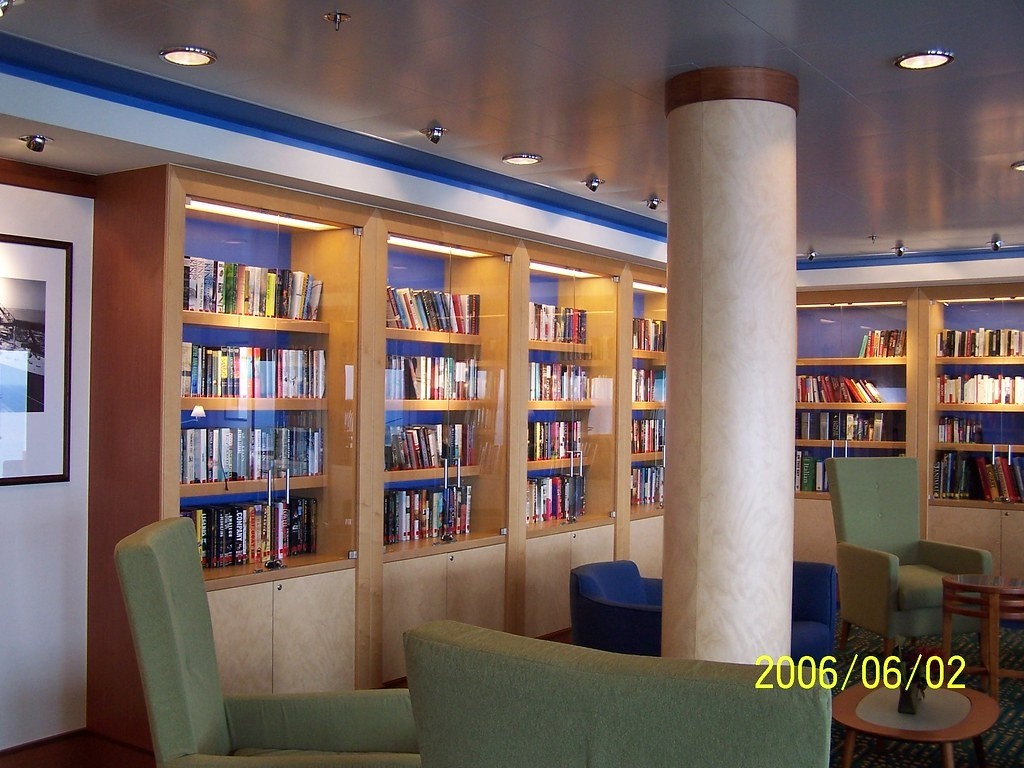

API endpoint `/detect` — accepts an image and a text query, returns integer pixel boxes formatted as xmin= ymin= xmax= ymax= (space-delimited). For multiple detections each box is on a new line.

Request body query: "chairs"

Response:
xmin=403 ymin=620 xmax=831 ymax=767
xmin=825 ymin=457 xmax=993 ymax=671
xmin=114 ymin=517 xmax=421 ymax=767
xmin=791 ymin=561 xmax=838 ymax=669
xmin=570 ymin=560 xmax=662 ymax=657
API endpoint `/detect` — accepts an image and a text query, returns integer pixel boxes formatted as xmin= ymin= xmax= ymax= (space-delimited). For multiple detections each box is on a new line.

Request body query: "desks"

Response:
xmin=940 ymin=574 xmax=1024 ymax=699
xmin=831 ymin=678 xmax=999 ymax=768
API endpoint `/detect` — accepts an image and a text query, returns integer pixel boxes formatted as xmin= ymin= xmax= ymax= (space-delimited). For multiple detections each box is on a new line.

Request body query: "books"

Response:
xmin=180 ymin=496 xmax=317 ymax=569
xmin=384 ymin=484 xmax=470 ymax=547
xmin=795 ymin=412 xmax=895 ymax=441
xmin=795 ymin=450 xmax=829 ymax=491
xmin=937 ymin=327 xmax=1024 ymax=357
xmin=528 ymin=420 xmax=581 ymax=460
xmin=529 ymin=302 xmax=587 ymax=343
xmin=631 ymin=418 xmax=666 ymax=453
xmin=936 ymin=373 xmax=1024 ymax=403
xmin=529 ymin=362 xmax=590 ymax=401
xmin=181 ymin=343 xmax=326 ymax=398
xmin=386 ymin=354 xmax=477 ymax=400
xmin=796 ymin=375 xmax=885 ymax=403
xmin=181 ymin=426 xmax=324 ymax=484
xmin=526 ymin=475 xmax=585 ymax=523
xmin=384 ymin=423 xmax=475 ymax=471
xmin=632 ymin=367 xmax=666 ymax=403
xmin=631 ymin=466 xmax=664 ymax=505
xmin=934 ymin=452 xmax=1024 ymax=503
xmin=857 ymin=329 xmax=906 ymax=358
xmin=633 ymin=317 xmax=666 ymax=352
xmin=385 ymin=287 xmax=480 ymax=335
xmin=183 ymin=255 xmax=322 ymax=320
xmin=938 ymin=416 xmax=984 ymax=444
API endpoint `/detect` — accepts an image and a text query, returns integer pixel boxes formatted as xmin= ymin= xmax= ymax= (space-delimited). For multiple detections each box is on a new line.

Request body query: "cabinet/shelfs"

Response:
xmin=86 ymin=167 xmax=1024 ymax=752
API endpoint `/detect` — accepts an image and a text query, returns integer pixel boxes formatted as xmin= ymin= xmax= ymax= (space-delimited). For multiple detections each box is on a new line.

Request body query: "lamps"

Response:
xmin=580 ymin=178 xmax=606 ymax=193
xmin=420 ymin=128 xmax=447 ymax=144
xmin=18 ymin=135 xmax=54 ymax=153
xmin=642 ymin=198 xmax=664 ymax=210
xmin=891 ymin=246 xmax=909 ymax=257
xmin=805 ymin=251 xmax=819 ymax=262
xmin=986 ymin=240 xmax=1004 ymax=251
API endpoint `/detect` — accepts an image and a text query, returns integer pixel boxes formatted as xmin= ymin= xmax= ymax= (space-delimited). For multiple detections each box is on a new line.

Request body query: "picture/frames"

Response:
xmin=0 ymin=233 xmax=73 ymax=487
